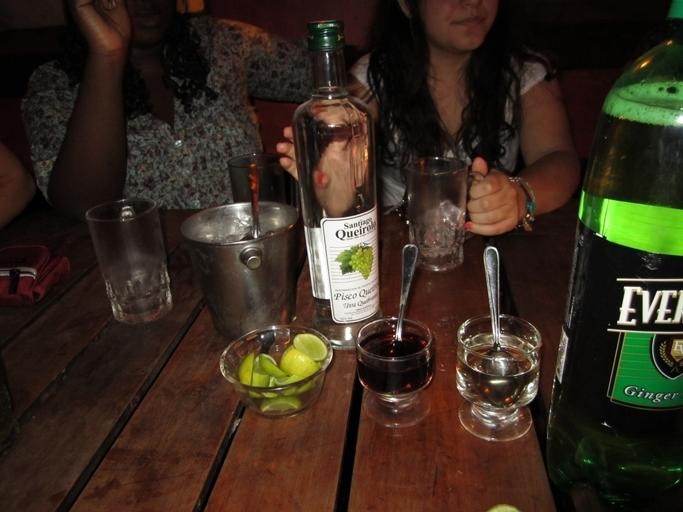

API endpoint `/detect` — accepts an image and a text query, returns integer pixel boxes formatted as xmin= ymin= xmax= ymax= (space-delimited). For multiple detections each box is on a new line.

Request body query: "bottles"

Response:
xmin=289 ymin=20 xmax=383 ymax=352
xmin=541 ymin=1 xmax=682 ymax=512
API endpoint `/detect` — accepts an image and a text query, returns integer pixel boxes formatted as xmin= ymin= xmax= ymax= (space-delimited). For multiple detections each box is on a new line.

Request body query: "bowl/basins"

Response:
xmin=218 ymin=323 xmax=335 ymax=418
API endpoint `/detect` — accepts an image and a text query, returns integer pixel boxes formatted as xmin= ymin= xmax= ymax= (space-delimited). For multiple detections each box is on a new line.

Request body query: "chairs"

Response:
xmin=549 ymin=67 xmax=629 ymax=199
xmin=243 ymin=93 xmax=313 ymax=214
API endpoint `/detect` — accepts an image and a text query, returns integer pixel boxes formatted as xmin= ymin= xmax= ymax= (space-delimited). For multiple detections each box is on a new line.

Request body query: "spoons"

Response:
xmin=477 ymin=247 xmax=521 ymax=408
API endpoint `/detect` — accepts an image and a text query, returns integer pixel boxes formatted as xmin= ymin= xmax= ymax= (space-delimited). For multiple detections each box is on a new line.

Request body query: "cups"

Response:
xmin=226 ymin=153 xmax=286 ymax=205
xmin=83 ymin=199 xmax=175 ymax=328
xmin=406 ymin=157 xmax=484 ymax=275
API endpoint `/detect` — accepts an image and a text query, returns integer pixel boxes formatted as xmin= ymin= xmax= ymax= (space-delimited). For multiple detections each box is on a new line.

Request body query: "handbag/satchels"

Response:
xmin=0 ymin=241 xmax=71 ymax=308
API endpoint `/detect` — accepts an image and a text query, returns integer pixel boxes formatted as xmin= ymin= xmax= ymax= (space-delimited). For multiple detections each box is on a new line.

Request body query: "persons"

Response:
xmin=22 ymin=1 xmax=313 ymax=219
xmin=275 ymin=0 xmax=582 ymax=237
xmin=1 ymin=147 xmax=34 ymax=227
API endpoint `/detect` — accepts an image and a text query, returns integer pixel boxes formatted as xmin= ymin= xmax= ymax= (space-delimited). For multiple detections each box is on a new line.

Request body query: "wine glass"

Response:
xmin=354 ymin=317 xmax=435 ymax=427
xmin=456 ymin=312 xmax=544 ymax=443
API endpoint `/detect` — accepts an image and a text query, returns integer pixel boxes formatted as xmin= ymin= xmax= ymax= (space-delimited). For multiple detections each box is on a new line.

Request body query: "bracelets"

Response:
xmin=507 ymin=176 xmax=537 ymax=233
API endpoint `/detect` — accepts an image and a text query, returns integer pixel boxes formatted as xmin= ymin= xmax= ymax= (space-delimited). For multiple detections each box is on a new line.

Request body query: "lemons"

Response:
xmin=237 ymin=333 xmax=327 ymax=413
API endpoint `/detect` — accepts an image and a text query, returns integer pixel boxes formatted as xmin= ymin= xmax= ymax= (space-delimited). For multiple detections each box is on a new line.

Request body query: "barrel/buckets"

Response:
xmin=181 ymin=199 xmax=301 ymax=343
